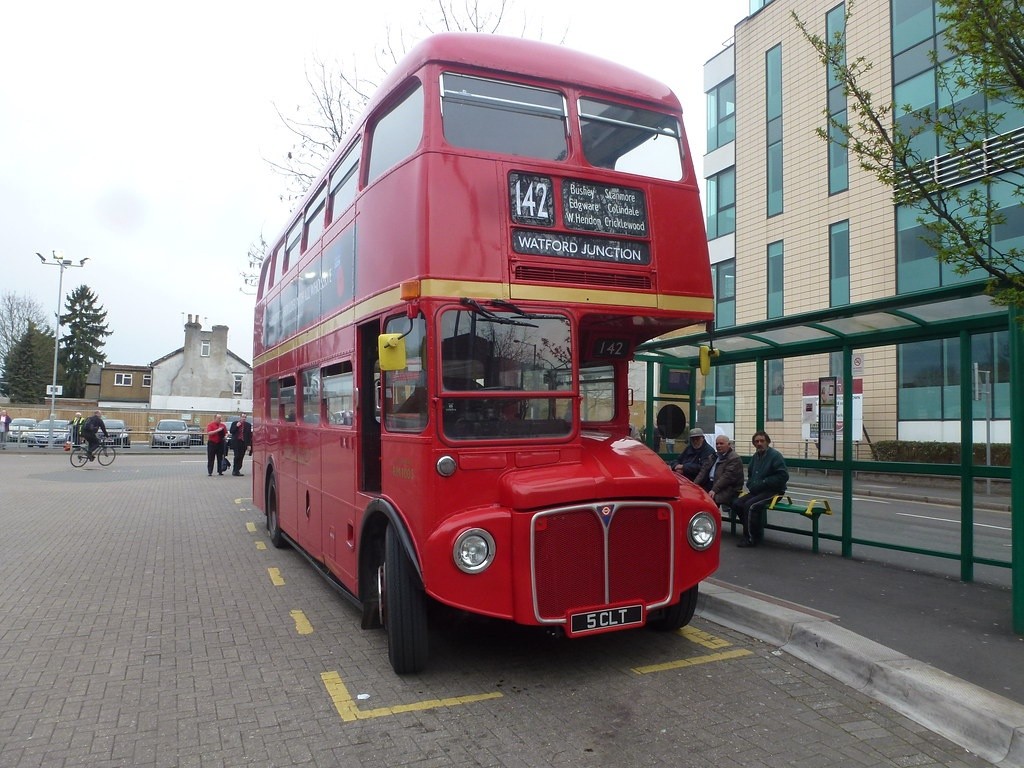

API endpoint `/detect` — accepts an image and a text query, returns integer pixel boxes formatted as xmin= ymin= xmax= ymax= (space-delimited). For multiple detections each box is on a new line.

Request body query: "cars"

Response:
xmin=225 ymin=422 xmax=233 ymax=443
xmin=8 ymin=418 xmax=37 ymax=442
xmin=330 ymin=411 xmax=354 ymax=425
xmin=187 ymin=425 xmax=204 ymax=445
xmin=28 ymin=420 xmax=71 ymax=448
xmin=229 ymin=416 xmax=253 ymax=433
xmin=150 ymin=420 xmax=191 ymax=448
xmin=95 ymin=420 xmax=133 ymax=448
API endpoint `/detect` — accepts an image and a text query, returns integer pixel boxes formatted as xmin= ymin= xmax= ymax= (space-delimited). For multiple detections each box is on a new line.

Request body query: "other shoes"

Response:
xmin=208 ymin=473 xmax=212 ymax=476
xmin=85 ymin=452 xmax=94 ymax=461
xmin=232 ymin=472 xmax=244 ymax=476
xmin=3 ymin=448 xmax=5 ymax=450
xmin=219 ymin=473 xmax=223 ymax=475
xmin=737 ymin=536 xmax=763 ymax=548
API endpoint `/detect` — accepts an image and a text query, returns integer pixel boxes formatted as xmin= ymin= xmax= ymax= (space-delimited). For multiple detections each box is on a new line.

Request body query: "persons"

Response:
xmin=0 ymin=410 xmax=12 ymax=450
xmin=693 ymin=434 xmax=744 ymax=504
xmin=80 ymin=409 xmax=111 ymax=461
xmin=733 ymin=431 xmax=790 ymax=549
xmin=668 ymin=427 xmax=716 ymax=481
xmin=229 ymin=412 xmax=252 ymax=477
xmin=206 ymin=413 xmax=229 ymax=476
xmin=628 ymin=409 xmax=641 ymax=442
xmin=66 ymin=412 xmax=85 ymax=451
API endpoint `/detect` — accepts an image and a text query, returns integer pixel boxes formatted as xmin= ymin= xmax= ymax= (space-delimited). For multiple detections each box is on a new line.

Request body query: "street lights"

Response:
xmin=36 ymin=251 xmax=90 ymax=449
xmin=513 ymin=339 xmax=536 ymax=370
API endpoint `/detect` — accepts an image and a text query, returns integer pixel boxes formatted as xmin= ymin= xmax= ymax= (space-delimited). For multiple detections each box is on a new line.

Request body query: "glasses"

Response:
xmin=242 ymin=416 xmax=246 ymax=418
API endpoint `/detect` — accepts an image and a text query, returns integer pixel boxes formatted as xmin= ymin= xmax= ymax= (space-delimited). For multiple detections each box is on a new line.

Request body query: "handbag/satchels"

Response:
xmin=84 ymin=422 xmax=98 ymax=433
xmin=222 ymin=455 xmax=231 ymax=471
xmin=227 ymin=439 xmax=233 ymax=449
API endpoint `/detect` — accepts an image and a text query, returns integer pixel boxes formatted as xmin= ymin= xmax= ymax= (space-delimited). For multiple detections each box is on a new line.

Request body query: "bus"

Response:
xmin=252 ymin=32 xmax=723 ymax=676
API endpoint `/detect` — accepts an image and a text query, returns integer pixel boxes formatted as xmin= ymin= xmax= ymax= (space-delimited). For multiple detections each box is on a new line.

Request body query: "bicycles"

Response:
xmin=70 ymin=435 xmax=116 ymax=467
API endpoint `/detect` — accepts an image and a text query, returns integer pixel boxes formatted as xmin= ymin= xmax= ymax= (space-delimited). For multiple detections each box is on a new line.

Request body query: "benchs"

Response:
xmin=735 ymin=493 xmax=831 ymax=555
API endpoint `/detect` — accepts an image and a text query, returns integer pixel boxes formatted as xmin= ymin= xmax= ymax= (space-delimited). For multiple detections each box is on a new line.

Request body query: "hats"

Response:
xmin=689 ymin=427 xmax=703 ymax=437
xmin=76 ymin=412 xmax=81 ymax=415
